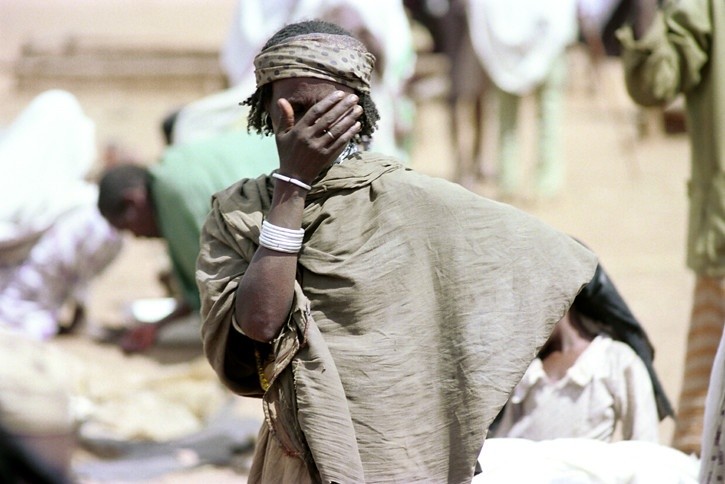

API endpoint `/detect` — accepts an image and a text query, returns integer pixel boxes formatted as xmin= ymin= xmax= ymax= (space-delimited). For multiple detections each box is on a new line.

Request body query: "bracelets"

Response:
xmin=258 ymin=220 xmax=305 ymax=254
xmin=272 ymin=172 xmax=311 ymax=192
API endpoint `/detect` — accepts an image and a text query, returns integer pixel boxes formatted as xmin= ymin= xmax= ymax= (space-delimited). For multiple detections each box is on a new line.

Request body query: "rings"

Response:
xmin=327 ymin=131 xmax=334 ymax=139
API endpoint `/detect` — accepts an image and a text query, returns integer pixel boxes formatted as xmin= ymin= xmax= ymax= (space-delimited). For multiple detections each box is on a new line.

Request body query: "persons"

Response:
xmin=438 ymin=0 xmax=566 ymax=194
xmin=616 ymin=0 xmax=725 ymax=280
xmin=96 ymin=122 xmax=279 ymax=359
xmin=486 ymin=282 xmax=661 ymax=443
xmin=196 ymin=20 xmax=675 ymax=484
